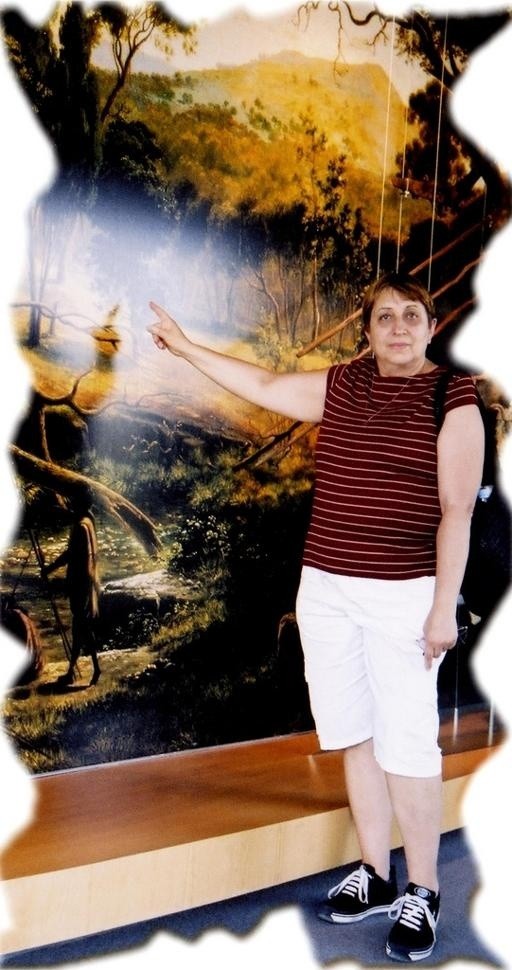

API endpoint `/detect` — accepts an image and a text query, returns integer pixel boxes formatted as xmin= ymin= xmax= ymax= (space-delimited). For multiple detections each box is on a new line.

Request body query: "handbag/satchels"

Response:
xmin=462 ymin=492 xmax=512 ymax=620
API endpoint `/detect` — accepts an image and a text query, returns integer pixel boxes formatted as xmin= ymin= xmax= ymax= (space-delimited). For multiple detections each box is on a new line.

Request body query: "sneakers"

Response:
xmin=316 ymin=865 xmax=397 ymax=924
xmin=388 ymin=883 xmax=439 ymax=961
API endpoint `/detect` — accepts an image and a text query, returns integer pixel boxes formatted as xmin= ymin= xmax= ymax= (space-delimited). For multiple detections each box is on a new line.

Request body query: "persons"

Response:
xmin=147 ymin=271 xmax=485 ymax=963
